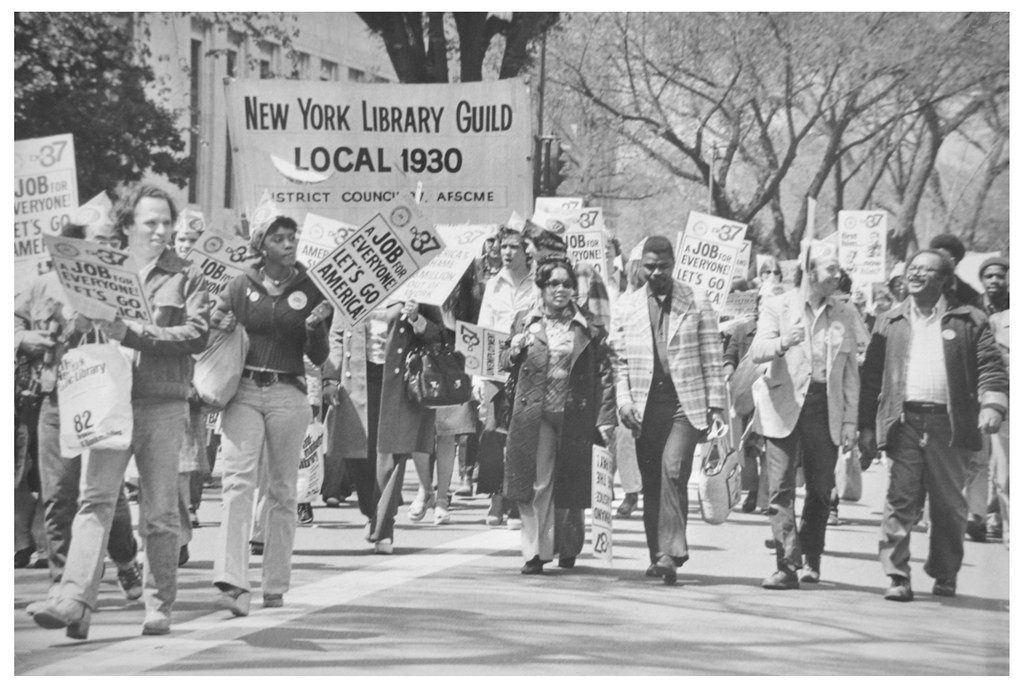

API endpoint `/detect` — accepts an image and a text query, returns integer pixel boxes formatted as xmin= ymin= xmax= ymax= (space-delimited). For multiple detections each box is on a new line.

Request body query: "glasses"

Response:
xmin=543 ymin=279 xmax=574 ymax=288
xmin=906 ymin=265 xmax=943 ymax=275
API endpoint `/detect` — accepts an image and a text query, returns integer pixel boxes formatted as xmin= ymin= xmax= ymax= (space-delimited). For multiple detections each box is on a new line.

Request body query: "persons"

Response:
xmin=14 ymin=184 xmax=1009 ymax=638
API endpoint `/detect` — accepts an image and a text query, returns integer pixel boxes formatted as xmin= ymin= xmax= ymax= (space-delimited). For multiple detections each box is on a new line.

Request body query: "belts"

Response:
xmin=807 ymin=382 xmax=825 ymax=392
xmin=241 ymin=367 xmax=309 ymax=395
xmin=901 ymin=401 xmax=948 ymax=415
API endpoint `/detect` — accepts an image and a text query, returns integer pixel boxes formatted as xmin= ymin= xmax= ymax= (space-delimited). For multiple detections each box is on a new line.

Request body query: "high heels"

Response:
xmin=143 ymin=611 xmax=170 ymax=634
xmin=34 ymin=598 xmax=91 ymax=639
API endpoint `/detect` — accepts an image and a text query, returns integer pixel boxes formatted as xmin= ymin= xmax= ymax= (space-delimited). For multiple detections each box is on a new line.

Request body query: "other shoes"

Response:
xmin=127 ymin=470 xmax=1006 ymax=600
xmin=262 ymin=593 xmax=282 ymax=607
xmin=29 ymin=557 xmax=49 ymax=569
xmin=117 ymin=561 xmax=144 ymax=600
xmin=178 ymin=543 xmax=189 ymax=565
xmin=15 ymin=545 xmax=36 ymax=568
xmin=209 ymin=586 xmax=250 ymax=615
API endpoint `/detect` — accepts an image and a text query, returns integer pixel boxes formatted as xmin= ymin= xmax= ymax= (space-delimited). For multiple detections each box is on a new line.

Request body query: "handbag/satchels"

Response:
xmin=191 ymin=320 xmax=251 ymax=406
xmin=404 ymin=325 xmax=472 ymax=407
xmin=834 ymin=439 xmax=863 ymax=502
xmin=56 ymin=322 xmax=137 ymax=457
xmin=296 ymin=418 xmax=325 ymax=504
xmin=698 ymin=419 xmax=741 ymax=524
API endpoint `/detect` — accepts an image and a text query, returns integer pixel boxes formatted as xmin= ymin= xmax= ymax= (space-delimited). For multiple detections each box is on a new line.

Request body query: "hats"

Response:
xmin=250 ymin=215 xmax=283 ymax=251
xmin=978 ymin=256 xmax=1009 ymax=281
